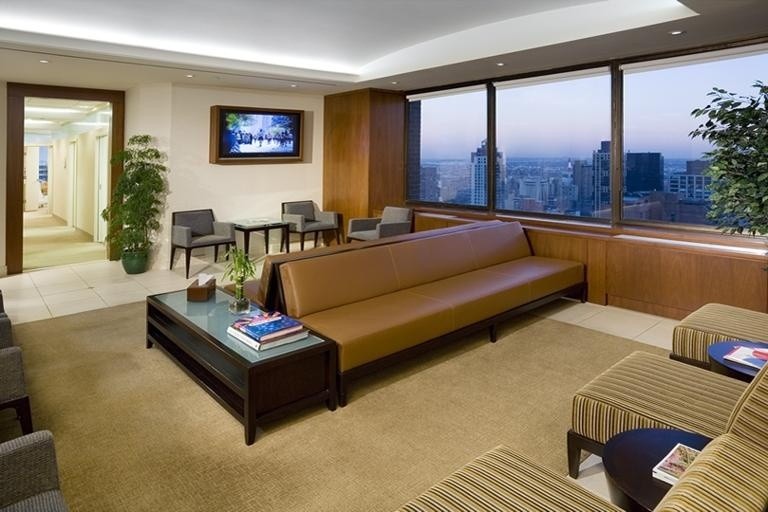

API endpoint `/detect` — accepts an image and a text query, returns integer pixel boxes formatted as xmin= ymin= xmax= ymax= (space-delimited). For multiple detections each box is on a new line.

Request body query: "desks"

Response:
xmin=143 ymin=285 xmax=339 ymax=446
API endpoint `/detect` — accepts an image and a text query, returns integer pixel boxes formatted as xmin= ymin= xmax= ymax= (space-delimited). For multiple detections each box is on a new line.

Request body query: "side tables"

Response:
xmin=232 ymin=218 xmax=290 ymax=263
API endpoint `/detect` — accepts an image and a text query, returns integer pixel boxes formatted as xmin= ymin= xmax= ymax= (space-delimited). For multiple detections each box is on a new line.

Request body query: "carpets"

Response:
xmin=10 ymin=286 xmax=675 ymax=509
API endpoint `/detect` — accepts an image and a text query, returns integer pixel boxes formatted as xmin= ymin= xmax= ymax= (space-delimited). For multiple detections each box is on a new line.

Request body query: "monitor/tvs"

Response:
xmin=219 ymin=109 xmax=302 ymax=160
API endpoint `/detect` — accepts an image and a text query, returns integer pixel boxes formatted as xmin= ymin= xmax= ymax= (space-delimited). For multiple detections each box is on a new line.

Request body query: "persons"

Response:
xmin=233 ymin=128 xmax=294 ymax=146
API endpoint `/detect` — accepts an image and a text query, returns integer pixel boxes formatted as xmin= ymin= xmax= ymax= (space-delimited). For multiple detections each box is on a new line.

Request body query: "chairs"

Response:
xmin=169 ymin=208 xmax=236 ymax=280
xmin=278 ymin=200 xmax=342 ymax=253
xmin=346 ymin=205 xmax=415 ymax=244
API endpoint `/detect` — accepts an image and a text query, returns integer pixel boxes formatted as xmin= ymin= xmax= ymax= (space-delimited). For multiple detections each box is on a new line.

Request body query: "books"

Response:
xmin=651 ymin=442 xmax=701 ymax=487
xmin=723 ymin=344 xmax=768 ymax=370
xmin=229 ymin=309 xmax=306 ymax=342
xmin=227 ymin=324 xmax=310 ymax=352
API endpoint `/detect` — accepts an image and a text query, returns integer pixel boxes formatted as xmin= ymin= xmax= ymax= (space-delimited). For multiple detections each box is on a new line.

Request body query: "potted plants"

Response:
xmin=223 ymin=248 xmax=255 ymax=311
xmin=97 ymin=130 xmax=175 ymax=276
